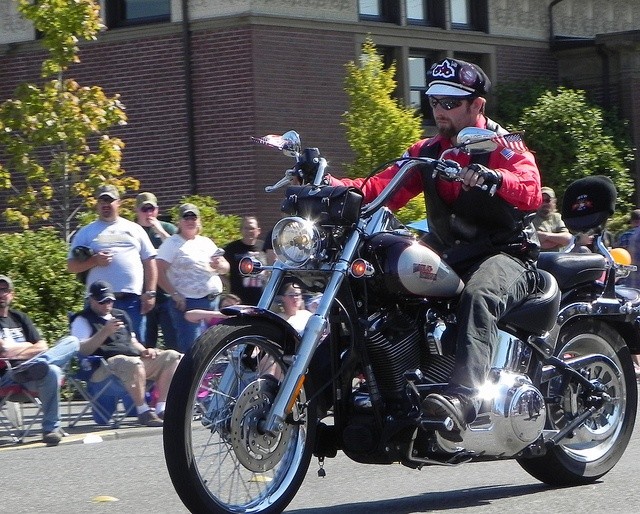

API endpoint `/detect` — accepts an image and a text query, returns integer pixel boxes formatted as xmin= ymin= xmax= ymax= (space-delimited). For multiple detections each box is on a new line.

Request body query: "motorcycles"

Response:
xmin=162 ymin=128 xmax=640 ymax=513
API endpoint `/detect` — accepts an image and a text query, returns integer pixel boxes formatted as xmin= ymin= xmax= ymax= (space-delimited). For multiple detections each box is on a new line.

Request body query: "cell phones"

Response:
xmin=112 ymin=312 xmax=129 ymax=331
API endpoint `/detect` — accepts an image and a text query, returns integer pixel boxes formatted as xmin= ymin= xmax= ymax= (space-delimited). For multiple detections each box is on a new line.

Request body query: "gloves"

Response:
xmin=465 ymin=163 xmax=504 ymax=189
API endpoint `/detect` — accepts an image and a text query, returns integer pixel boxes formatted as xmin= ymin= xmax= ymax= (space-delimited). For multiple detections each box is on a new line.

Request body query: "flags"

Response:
xmin=260 ymin=130 xmax=299 ymax=150
xmin=490 ymin=133 xmax=528 ymax=156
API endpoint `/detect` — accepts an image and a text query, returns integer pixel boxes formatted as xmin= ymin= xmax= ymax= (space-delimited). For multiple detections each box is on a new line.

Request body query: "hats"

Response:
xmin=96 ymin=184 xmax=120 ymax=199
xmin=541 ymin=187 xmax=556 ymax=198
xmin=88 ymin=280 xmax=116 ymax=303
xmin=136 ymin=192 xmax=158 ymax=209
xmin=426 ymin=57 xmax=491 ymax=96
xmin=179 ymin=203 xmax=198 ymax=218
xmin=0 ymin=275 xmax=14 ymax=289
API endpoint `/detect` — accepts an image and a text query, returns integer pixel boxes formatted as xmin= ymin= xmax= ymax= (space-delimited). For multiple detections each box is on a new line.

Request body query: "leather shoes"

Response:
xmin=423 ymin=393 xmax=472 ymax=442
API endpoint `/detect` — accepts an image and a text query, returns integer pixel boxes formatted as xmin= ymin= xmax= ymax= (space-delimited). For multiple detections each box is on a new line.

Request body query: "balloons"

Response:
xmin=609 ymin=248 xmax=631 ymax=266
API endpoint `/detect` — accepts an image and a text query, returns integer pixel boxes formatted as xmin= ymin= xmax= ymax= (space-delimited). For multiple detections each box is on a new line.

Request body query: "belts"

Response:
xmin=186 ymin=294 xmax=220 ymax=301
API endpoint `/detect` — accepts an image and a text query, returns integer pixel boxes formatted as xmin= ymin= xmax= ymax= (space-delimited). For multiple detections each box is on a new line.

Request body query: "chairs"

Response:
xmin=0 ymin=379 xmax=65 ymax=444
xmin=68 ymin=310 xmax=155 ymax=432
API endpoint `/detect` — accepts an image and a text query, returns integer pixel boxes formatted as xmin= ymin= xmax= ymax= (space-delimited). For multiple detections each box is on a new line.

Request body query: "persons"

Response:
xmin=184 ymin=293 xmax=245 ymax=398
xmin=615 ymin=209 xmax=640 ymax=290
xmin=0 ymin=275 xmax=80 ymax=447
xmin=223 ymin=217 xmax=271 ymax=306
xmin=68 ymin=185 xmax=157 ymax=424
xmin=531 ymin=187 xmax=571 ymax=253
xmin=274 ymin=283 xmax=314 ymax=338
xmin=572 ymin=228 xmax=594 ymax=253
xmin=158 ymin=203 xmax=231 ymax=355
xmin=71 ymin=281 xmax=184 ymax=427
xmin=289 ymin=57 xmax=543 ymax=440
xmin=132 ymin=191 xmax=180 ymax=352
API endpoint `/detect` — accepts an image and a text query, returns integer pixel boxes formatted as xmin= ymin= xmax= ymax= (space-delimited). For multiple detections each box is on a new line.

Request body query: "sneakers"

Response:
xmin=43 ymin=432 xmax=61 ymax=446
xmin=13 ymin=361 xmax=49 ymax=383
xmin=138 ymin=408 xmax=163 ymax=427
xmin=157 ymin=409 xmax=165 ymax=419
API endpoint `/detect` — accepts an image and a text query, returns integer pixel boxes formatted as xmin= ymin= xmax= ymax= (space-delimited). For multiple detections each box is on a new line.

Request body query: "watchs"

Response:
xmin=145 ymin=290 xmax=156 ymax=297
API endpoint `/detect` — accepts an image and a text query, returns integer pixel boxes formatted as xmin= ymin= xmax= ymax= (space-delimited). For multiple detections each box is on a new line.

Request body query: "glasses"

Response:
xmin=100 ymin=197 xmax=115 ymax=202
xmin=542 ymin=198 xmax=553 ymax=203
xmin=99 ymin=299 xmax=112 ymax=304
xmin=0 ymin=289 xmax=12 ymax=294
xmin=140 ymin=206 xmax=155 ymax=212
xmin=183 ymin=214 xmax=196 ymax=220
xmin=428 ymin=97 xmax=474 ymax=110
xmin=288 ymin=292 xmax=301 ymax=297
